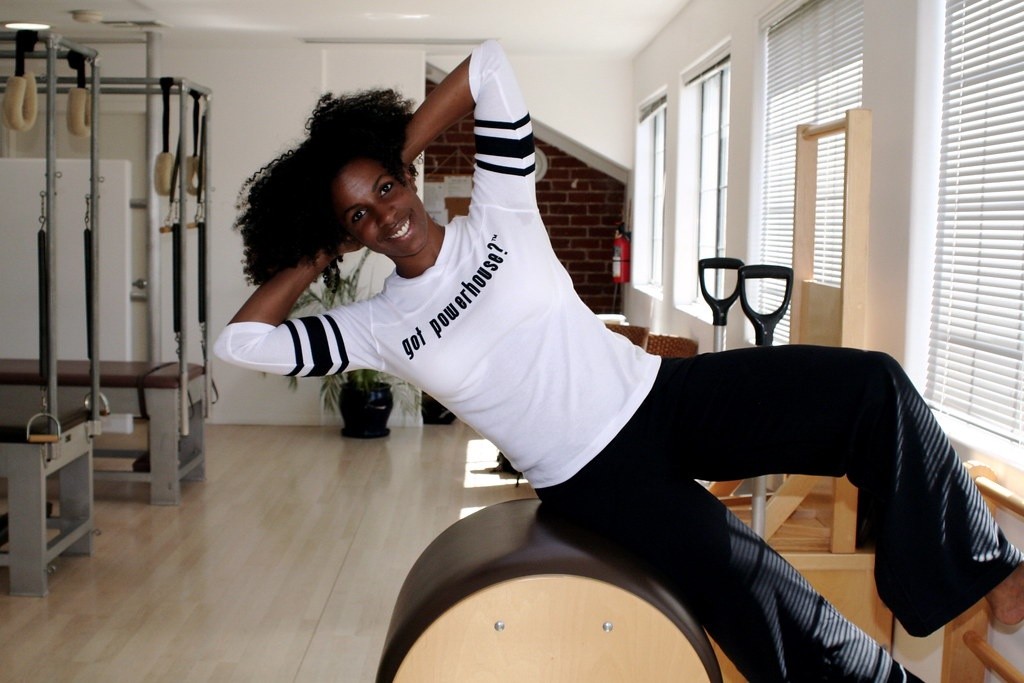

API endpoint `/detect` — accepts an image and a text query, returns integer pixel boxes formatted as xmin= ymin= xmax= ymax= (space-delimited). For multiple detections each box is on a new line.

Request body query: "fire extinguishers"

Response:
xmin=611 ymin=221 xmax=631 ymax=284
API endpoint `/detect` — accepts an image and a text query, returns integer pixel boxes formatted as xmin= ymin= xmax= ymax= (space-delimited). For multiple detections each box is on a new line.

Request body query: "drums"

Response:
xmin=373 ymin=495 xmax=725 ymax=683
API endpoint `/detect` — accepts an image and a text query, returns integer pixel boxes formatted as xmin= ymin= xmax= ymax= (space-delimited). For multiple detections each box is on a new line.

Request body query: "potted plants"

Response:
xmin=261 ymin=244 xmax=429 ymax=440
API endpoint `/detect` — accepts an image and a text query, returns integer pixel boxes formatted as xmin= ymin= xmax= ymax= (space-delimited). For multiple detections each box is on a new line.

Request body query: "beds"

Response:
xmin=0 ymin=400 xmax=101 ymax=599
xmin=0 ymin=358 xmax=208 ymax=503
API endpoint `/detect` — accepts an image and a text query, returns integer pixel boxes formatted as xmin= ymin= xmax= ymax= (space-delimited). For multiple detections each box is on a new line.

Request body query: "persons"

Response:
xmin=212 ymin=38 xmax=1024 ymax=683
